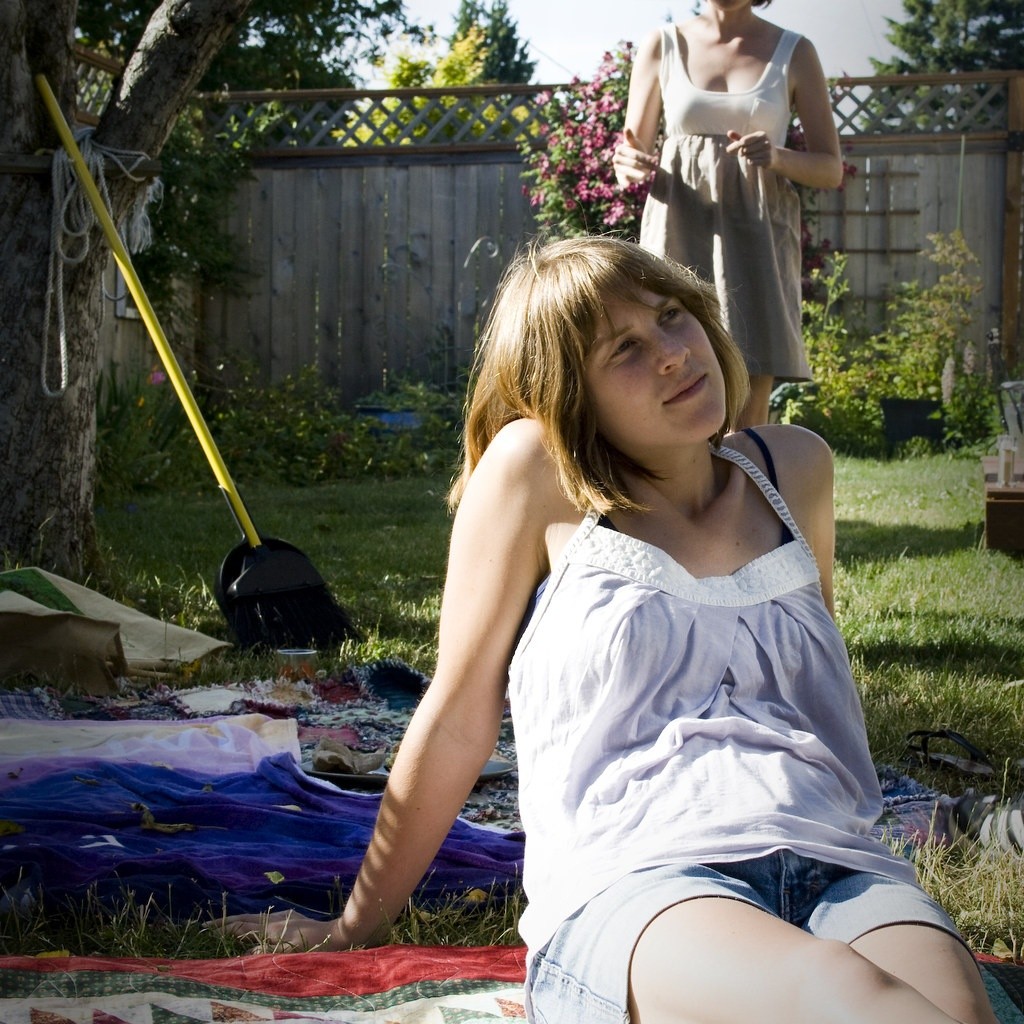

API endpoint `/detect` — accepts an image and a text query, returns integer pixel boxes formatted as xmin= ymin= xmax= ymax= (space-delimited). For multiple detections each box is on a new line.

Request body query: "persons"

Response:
xmin=613 ymin=0 xmax=842 ymax=438
xmin=201 ymin=231 xmax=999 ymax=1024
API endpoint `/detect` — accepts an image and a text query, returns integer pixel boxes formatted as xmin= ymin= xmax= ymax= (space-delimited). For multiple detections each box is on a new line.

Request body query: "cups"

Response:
xmin=278 ymin=650 xmax=318 ymax=680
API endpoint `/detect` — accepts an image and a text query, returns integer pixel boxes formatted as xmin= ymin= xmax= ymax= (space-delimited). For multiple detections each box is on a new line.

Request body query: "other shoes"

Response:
xmin=947 ymin=793 xmax=1024 ymax=873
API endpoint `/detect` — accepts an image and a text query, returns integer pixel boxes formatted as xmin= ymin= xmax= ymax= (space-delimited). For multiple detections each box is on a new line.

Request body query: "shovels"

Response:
xmin=31 ymin=61 xmax=356 ymax=657
